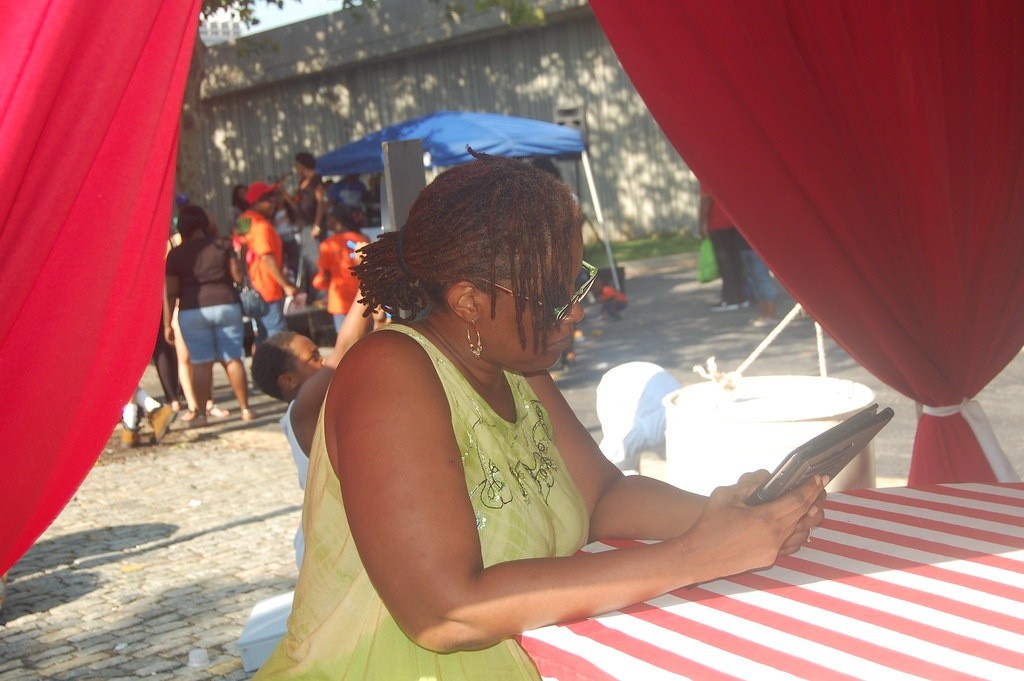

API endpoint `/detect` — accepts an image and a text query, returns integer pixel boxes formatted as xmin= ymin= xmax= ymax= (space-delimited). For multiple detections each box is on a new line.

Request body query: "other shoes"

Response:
xmin=121 ymin=423 xmax=141 ymax=448
xmin=240 ymin=409 xmax=256 ymax=421
xmin=148 ymin=403 xmax=177 ymax=443
xmin=712 ymin=299 xmax=748 ymax=311
xmin=205 ymin=405 xmax=231 ymax=418
xmin=169 ymin=408 xmax=208 ymax=430
xmin=170 ymin=398 xmax=183 ymax=414
xmin=753 ymin=315 xmax=779 ymax=327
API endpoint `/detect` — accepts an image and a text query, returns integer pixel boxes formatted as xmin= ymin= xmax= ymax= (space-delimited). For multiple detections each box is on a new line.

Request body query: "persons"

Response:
xmin=321 ymin=174 xmax=380 ymax=227
xmin=312 ymin=204 xmax=373 ymax=333
xmin=698 ymin=183 xmax=754 ymax=313
xmin=229 ymin=182 xmax=301 ymax=395
xmin=226 ymin=184 xmax=256 ymax=290
xmin=247 ymin=146 xmax=832 ymax=681
xmin=251 ymin=242 xmax=392 ymax=570
xmin=277 ymin=150 xmax=329 ymax=305
xmin=273 ymin=182 xmax=301 ymax=274
xmin=119 ymin=194 xmax=257 ymax=449
xmin=592 ymin=278 xmax=628 ymax=321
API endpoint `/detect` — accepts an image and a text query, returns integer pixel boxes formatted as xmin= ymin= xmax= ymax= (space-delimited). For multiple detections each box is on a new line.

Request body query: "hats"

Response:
xmin=296 ymin=152 xmax=315 ymax=168
xmin=245 ymin=182 xmax=277 ymax=205
xmin=176 ymin=193 xmax=189 ymax=207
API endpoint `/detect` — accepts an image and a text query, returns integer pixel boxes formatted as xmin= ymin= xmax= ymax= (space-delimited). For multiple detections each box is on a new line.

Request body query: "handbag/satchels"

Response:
xmin=239 ymin=285 xmax=268 ymax=319
xmin=697 ymin=239 xmax=719 ymax=284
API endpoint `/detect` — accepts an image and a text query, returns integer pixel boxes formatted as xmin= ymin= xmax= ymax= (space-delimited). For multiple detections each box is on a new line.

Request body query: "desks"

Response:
xmin=515 ymin=484 xmax=1023 ymax=681
xmin=286 ymin=310 xmax=338 ymax=345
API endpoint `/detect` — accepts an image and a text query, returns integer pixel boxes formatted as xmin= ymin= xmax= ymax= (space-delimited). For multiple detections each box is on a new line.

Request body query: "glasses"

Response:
xmin=483 ymin=255 xmax=598 ymax=324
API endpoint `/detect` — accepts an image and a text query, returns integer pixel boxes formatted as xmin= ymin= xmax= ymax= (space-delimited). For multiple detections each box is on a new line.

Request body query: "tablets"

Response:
xmin=748 ymin=403 xmax=894 ymax=506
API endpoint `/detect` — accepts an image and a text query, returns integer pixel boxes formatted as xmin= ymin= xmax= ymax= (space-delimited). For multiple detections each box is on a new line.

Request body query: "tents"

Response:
xmin=317 ymin=109 xmax=622 ymax=292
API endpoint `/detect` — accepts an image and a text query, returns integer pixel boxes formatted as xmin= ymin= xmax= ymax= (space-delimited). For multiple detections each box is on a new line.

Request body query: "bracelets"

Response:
xmin=313 ymin=224 xmax=322 ymax=233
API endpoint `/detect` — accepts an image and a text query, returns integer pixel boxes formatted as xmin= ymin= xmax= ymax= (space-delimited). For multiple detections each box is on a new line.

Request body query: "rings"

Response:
xmin=806 ymin=528 xmax=812 ymax=543
